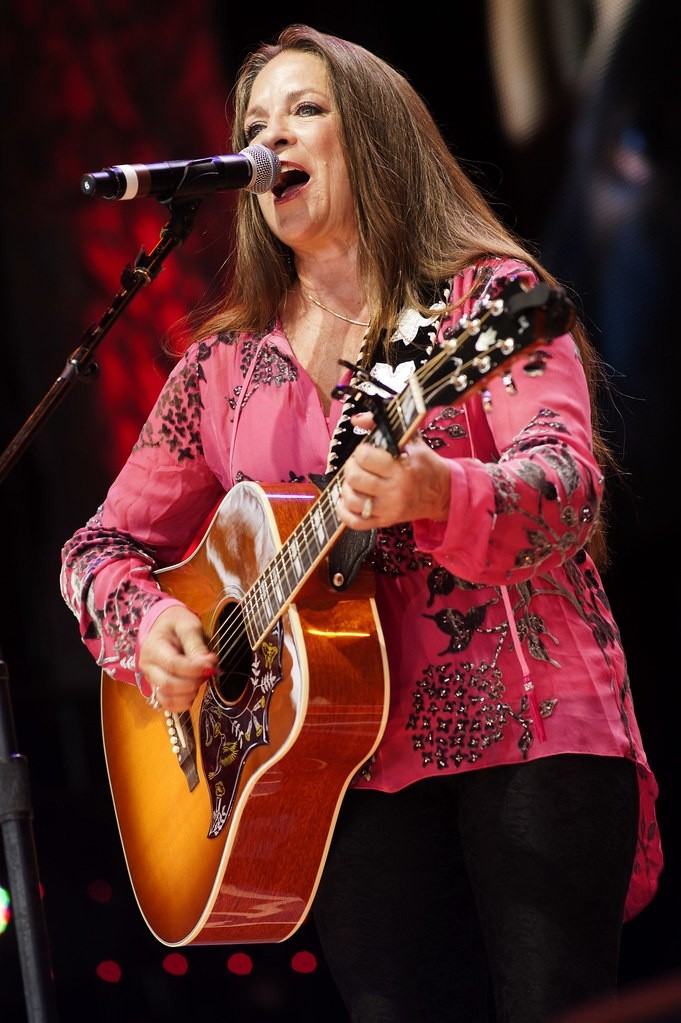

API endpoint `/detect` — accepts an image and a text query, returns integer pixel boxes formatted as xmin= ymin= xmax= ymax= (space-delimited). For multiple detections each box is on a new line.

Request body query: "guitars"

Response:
xmin=97 ymin=281 xmax=580 ymax=951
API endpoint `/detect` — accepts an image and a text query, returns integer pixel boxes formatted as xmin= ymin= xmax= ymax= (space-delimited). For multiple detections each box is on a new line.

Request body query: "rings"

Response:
xmin=361 ymin=497 xmax=375 ymax=519
xmin=146 ymin=693 xmax=163 ymax=711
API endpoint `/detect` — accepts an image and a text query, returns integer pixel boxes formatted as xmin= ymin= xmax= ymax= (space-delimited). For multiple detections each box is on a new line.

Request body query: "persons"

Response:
xmin=55 ymin=23 xmax=665 ymax=1023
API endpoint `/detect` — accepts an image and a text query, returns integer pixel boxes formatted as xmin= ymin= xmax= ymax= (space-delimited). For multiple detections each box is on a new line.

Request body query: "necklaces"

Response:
xmin=304 ymin=292 xmax=362 ymax=341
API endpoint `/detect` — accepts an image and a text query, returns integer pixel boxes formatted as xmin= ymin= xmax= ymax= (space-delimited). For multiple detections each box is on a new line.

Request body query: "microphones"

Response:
xmin=79 ymin=143 xmax=281 ymax=200
